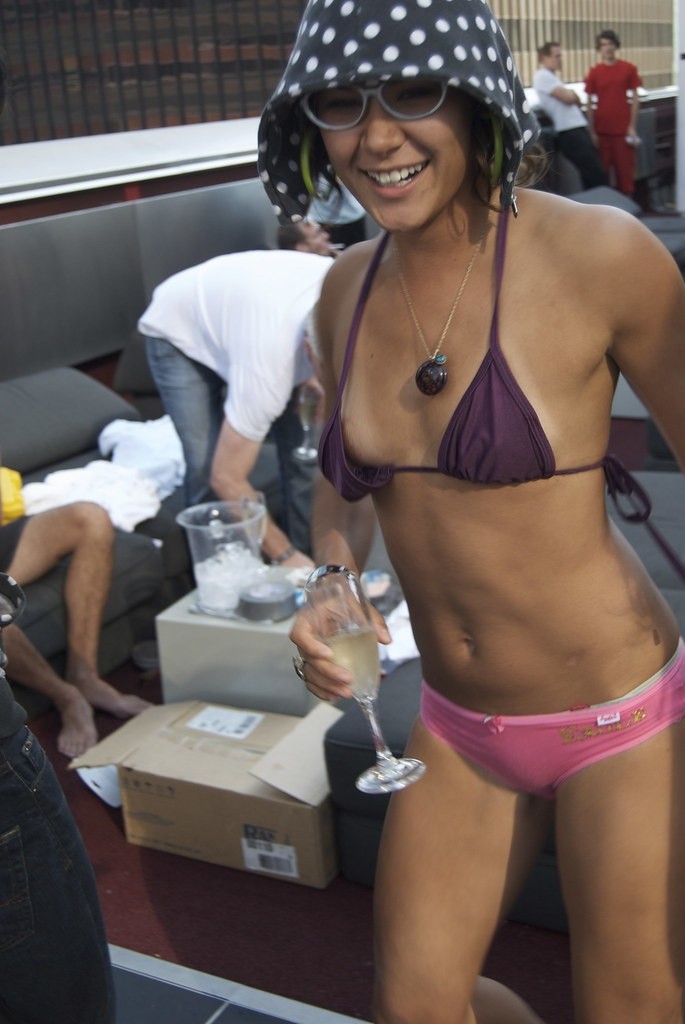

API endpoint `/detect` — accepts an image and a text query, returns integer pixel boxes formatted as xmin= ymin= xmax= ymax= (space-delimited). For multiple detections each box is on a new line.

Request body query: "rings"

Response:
xmin=291 ymin=655 xmax=308 ymax=682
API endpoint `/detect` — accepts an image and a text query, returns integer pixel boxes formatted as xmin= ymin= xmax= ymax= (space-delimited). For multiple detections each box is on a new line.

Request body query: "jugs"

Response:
xmin=175 ymin=493 xmax=266 ymax=617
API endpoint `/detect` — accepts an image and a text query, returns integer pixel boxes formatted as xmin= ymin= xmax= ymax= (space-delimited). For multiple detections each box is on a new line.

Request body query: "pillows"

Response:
xmin=1 ymin=363 xmax=141 ymax=473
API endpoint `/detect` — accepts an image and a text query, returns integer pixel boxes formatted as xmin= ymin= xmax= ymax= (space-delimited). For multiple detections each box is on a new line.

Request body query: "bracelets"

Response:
xmin=305 ymin=563 xmax=365 ymax=607
xmin=269 ymin=546 xmax=296 ymax=566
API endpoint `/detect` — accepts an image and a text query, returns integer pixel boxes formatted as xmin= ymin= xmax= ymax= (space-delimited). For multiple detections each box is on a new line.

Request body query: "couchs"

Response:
xmin=327 ymin=472 xmax=684 ymax=935
xmin=567 ymin=183 xmax=685 ymax=271
xmin=0 ymin=385 xmax=281 ymax=718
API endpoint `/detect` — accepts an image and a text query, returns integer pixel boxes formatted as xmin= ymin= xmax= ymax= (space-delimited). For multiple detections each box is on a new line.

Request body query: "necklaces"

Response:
xmin=391 ymin=188 xmax=497 ymax=395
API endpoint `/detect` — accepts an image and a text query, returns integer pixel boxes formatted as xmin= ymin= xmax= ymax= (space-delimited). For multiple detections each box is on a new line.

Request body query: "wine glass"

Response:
xmin=295 ymin=387 xmax=319 ymax=462
xmin=303 ymin=572 xmax=426 ymax=794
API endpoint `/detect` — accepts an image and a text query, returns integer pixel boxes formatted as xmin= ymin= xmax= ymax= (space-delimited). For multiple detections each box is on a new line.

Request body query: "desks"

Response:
xmin=151 ymin=586 xmax=344 ymax=722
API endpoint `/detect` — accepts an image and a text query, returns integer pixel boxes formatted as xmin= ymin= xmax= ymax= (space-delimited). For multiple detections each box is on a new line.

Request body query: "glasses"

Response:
xmin=298 ymin=75 xmax=447 ymax=131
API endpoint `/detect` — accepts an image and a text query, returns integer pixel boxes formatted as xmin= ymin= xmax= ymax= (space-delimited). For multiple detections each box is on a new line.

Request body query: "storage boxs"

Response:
xmin=69 ymin=701 xmax=349 ymax=889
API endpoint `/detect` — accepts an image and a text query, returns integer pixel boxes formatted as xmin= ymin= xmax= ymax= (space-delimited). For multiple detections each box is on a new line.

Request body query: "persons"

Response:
xmin=250 ymin=0 xmax=685 ymax=1024
xmin=135 ymin=249 xmax=334 ymax=601
xmin=533 ymin=31 xmax=643 ymax=199
xmin=0 ymin=467 xmax=157 ymax=759
xmin=278 ymin=163 xmax=370 ymax=264
